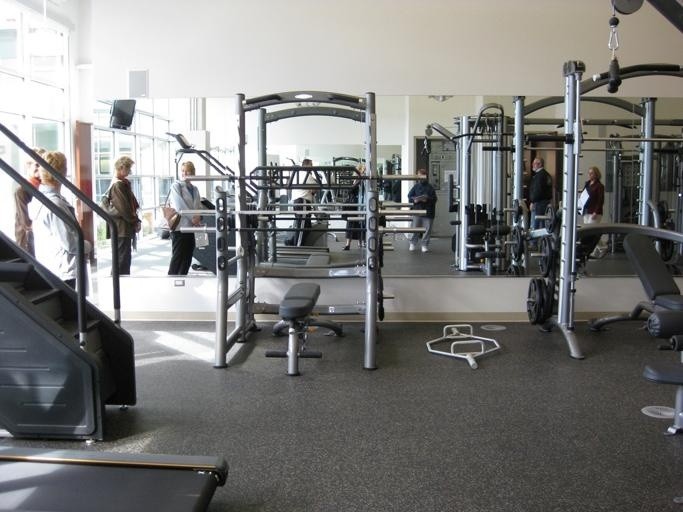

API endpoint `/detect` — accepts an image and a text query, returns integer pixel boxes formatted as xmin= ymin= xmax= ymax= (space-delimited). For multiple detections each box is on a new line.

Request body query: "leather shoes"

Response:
xmin=341 ymin=245 xmax=350 ymax=250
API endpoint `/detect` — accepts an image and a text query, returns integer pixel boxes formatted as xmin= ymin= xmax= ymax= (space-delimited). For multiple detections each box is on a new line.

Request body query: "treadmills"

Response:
xmin=0 ymin=442 xmax=228 ymax=511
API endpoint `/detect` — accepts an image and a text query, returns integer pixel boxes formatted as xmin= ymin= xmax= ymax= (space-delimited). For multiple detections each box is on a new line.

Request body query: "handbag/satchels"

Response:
xmin=162 ymin=181 xmax=181 ymax=232
xmin=98 ymin=179 xmax=121 ymax=218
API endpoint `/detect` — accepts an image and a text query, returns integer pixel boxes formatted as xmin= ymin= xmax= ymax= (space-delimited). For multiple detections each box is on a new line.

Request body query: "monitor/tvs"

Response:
xmin=110 ymin=99 xmax=136 ymax=130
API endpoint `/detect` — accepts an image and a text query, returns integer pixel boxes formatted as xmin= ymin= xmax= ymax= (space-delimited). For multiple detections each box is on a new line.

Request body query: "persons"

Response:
xmin=167 ymin=161 xmax=202 ymax=275
xmin=96 ymin=156 xmax=142 ymax=276
xmin=578 ymin=166 xmax=609 ymax=260
xmin=341 ymin=163 xmax=366 ymax=250
xmin=526 ymin=157 xmax=554 ymax=251
xmin=13 ymin=148 xmax=47 ymax=257
xmin=406 ymin=169 xmax=439 ymax=253
xmin=282 ymin=159 xmax=320 ymax=247
xmin=25 ymin=151 xmax=94 ymax=291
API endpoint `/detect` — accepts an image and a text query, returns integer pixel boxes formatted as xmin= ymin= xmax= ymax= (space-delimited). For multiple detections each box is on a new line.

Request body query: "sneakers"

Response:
xmin=409 ymin=243 xmax=417 ymax=251
xmin=420 ymin=244 xmax=429 ymax=252
